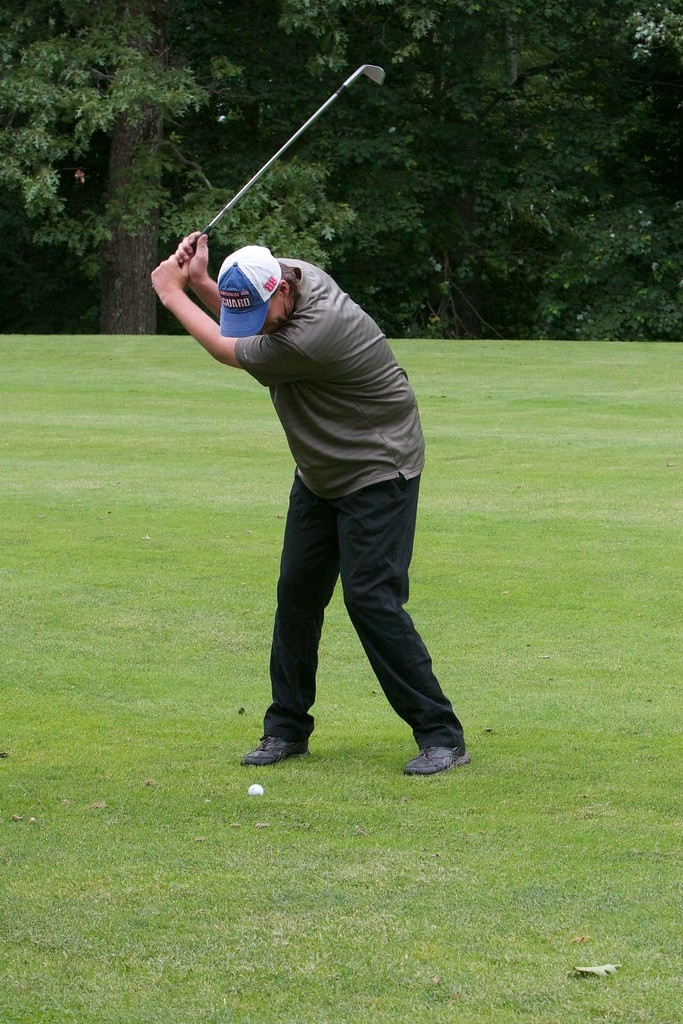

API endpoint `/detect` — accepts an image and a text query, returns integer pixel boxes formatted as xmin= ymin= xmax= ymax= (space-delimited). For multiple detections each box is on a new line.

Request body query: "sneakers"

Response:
xmin=240 ymin=737 xmax=309 ymax=767
xmin=404 ymin=747 xmax=471 ymax=775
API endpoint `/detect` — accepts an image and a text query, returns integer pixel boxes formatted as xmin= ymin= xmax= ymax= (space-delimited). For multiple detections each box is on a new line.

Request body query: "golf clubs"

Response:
xmin=178 ymin=63 xmax=388 ymax=268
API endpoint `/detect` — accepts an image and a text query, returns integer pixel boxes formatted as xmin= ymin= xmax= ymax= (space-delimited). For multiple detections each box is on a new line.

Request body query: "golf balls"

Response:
xmin=248 ymin=783 xmax=264 ymax=796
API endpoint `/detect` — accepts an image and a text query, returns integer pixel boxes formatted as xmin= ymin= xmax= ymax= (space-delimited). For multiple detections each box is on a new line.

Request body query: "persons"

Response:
xmin=150 ymin=231 xmax=470 ymax=775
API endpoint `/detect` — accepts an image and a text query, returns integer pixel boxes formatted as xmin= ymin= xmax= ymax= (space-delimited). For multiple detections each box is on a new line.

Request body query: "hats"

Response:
xmin=218 ymin=244 xmax=283 ymax=337
